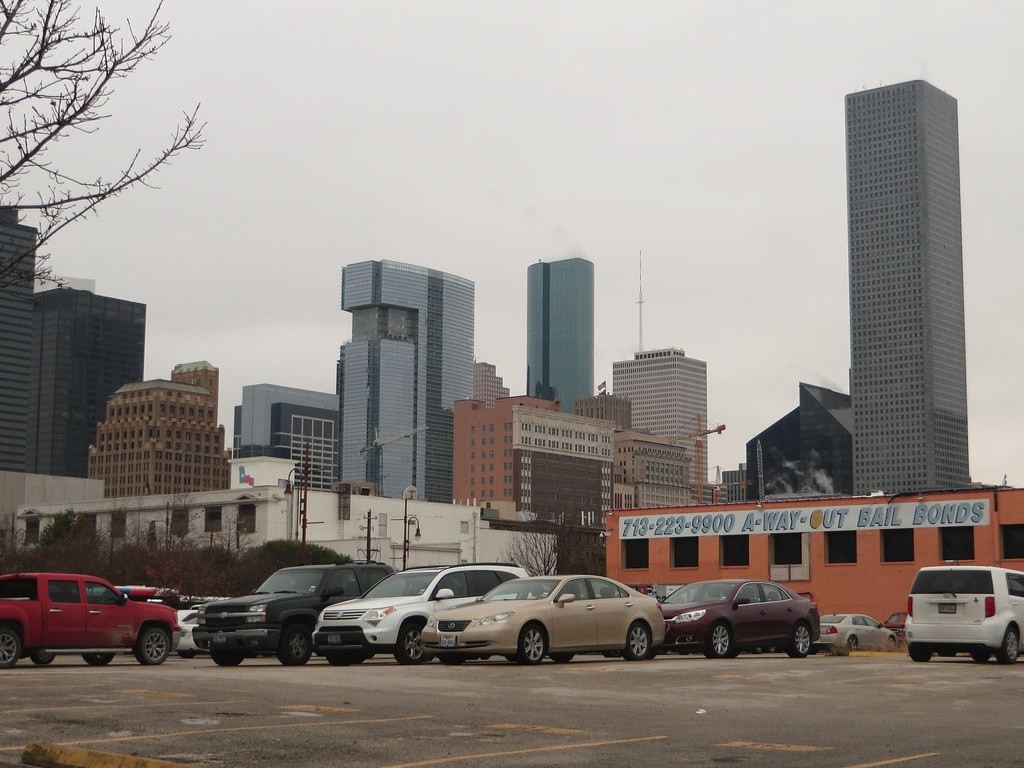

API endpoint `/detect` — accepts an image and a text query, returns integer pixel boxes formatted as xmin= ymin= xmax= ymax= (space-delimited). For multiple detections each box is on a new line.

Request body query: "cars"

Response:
xmin=419 ymin=575 xmax=666 ymax=665
xmin=174 ymin=612 xmax=210 ymax=658
xmin=808 ymin=613 xmax=899 ymax=656
xmin=649 ymin=579 xmax=822 ymax=659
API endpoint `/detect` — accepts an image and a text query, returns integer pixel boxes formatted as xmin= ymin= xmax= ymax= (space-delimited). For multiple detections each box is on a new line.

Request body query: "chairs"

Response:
xmin=741 ymin=588 xmax=758 ymax=604
xmin=563 ymin=582 xmax=582 ymax=602
xmin=707 ymin=589 xmax=722 ymax=602
xmin=528 ymin=585 xmax=546 ymax=600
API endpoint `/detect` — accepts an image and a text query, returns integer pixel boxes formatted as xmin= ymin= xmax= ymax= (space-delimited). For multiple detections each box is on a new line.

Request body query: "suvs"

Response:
xmin=191 ymin=558 xmax=395 ymax=667
xmin=312 ymin=562 xmax=532 ymax=665
xmin=884 ymin=612 xmax=909 ymax=652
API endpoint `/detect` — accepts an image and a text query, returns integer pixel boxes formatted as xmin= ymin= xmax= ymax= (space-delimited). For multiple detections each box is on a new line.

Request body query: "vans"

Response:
xmin=903 ymin=564 xmax=1024 ymax=664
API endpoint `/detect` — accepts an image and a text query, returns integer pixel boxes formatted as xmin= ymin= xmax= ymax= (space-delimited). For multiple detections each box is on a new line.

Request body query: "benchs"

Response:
xmin=582 ymin=587 xmax=617 ymax=599
xmin=752 ymin=591 xmax=778 ymax=602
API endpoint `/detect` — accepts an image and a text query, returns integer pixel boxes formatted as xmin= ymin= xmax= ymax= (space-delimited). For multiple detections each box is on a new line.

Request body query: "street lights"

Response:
xmin=283 ymin=467 xmax=303 ymax=541
xmin=407 ymin=517 xmax=422 ymax=559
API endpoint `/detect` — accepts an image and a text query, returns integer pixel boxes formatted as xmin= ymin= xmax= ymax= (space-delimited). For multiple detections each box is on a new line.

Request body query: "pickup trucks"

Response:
xmin=0 ymin=572 xmax=183 ymax=669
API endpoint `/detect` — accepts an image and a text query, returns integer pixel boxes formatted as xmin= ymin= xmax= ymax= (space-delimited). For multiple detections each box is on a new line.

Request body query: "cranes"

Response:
xmin=689 ymin=466 xmax=755 ymax=503
xmin=360 ymin=424 xmax=430 ymax=496
xmin=668 ymin=414 xmax=726 ymax=504
